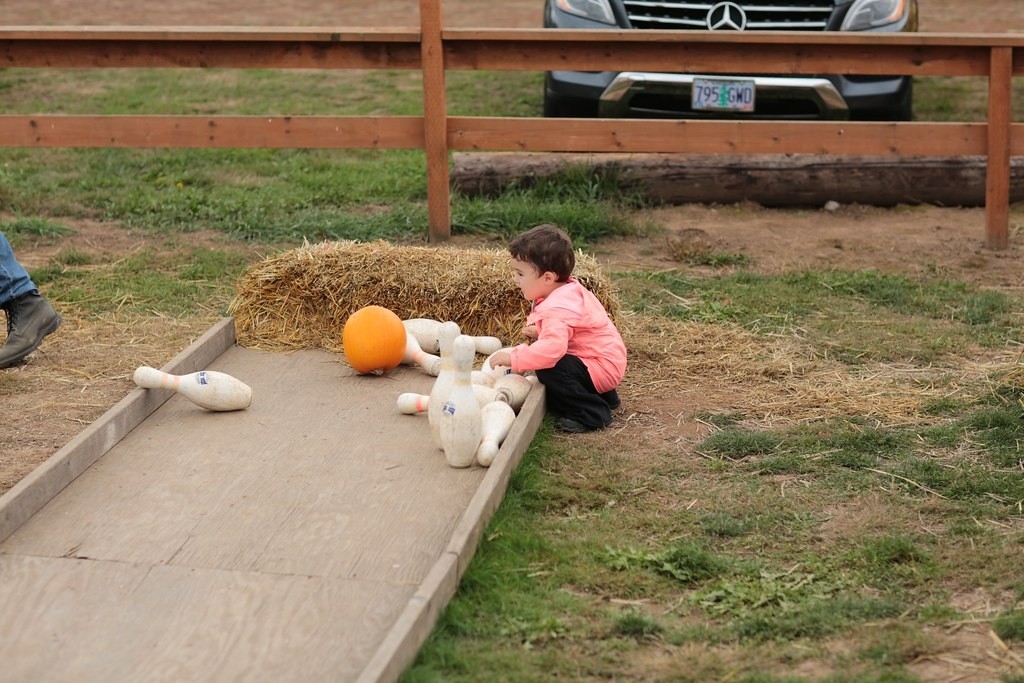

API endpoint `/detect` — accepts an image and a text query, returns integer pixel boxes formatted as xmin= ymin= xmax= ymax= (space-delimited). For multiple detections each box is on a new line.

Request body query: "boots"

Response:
xmin=0 ymin=294 xmax=63 ymax=368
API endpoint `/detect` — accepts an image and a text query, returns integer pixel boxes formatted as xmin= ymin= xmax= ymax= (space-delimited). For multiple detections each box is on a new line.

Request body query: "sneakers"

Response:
xmin=558 ymin=414 xmax=590 ymax=435
xmin=608 ymin=395 xmax=621 ymax=410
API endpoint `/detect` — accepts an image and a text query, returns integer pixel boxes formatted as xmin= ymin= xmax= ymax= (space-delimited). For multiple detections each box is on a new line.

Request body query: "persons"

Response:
xmin=489 ymin=224 xmax=627 ymax=433
xmin=1 ymin=233 xmax=62 ymax=369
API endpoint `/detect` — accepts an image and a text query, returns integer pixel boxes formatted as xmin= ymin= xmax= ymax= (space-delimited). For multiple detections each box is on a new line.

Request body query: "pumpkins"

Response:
xmin=342 ymin=306 xmax=406 ymax=376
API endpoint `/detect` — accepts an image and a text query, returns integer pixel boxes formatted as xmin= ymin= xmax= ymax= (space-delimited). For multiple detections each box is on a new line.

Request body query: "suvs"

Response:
xmin=543 ymin=0 xmax=918 ymax=122
